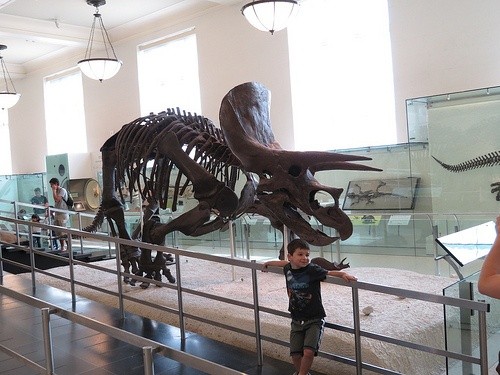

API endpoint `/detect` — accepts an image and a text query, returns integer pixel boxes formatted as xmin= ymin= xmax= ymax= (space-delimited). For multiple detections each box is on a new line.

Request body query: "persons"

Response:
xmin=50 ymin=177 xmax=72 ymax=252
xmin=17 ymin=209 xmax=28 ymax=225
xmin=478 ymin=178 xmax=500 ymax=375
xmin=260 ymin=238 xmax=357 ymax=375
xmin=27 ymin=214 xmax=43 ymax=249
xmin=45 ymin=206 xmax=59 ymax=250
xmin=30 ymin=188 xmax=50 ymax=248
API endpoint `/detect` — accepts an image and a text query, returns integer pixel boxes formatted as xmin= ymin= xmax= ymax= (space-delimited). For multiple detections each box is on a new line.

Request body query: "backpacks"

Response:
xmin=62 ymin=190 xmax=73 ymax=209
